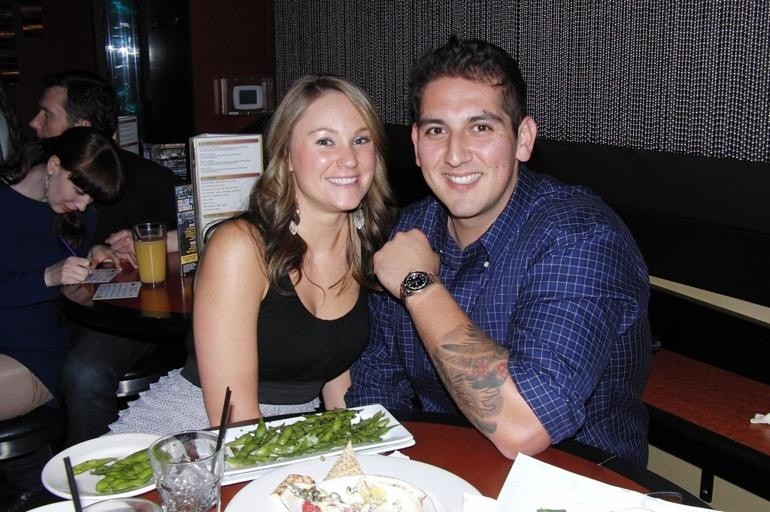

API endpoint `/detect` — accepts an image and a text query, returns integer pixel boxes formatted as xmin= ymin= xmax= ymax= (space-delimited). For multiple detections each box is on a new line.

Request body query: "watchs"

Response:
xmin=399 ymin=269 xmax=439 ymax=304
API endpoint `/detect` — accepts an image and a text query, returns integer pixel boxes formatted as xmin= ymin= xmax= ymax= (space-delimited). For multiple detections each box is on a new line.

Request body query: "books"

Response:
xmin=141 ymin=141 xmax=199 ymax=264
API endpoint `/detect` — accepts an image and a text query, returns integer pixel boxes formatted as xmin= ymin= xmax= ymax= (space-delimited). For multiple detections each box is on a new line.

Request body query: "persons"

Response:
xmin=29 ymin=69 xmax=183 ymax=255
xmin=2 ymin=129 xmax=137 ymax=422
xmin=342 ymin=33 xmax=652 ymax=473
xmin=104 ymin=74 xmax=395 ymax=434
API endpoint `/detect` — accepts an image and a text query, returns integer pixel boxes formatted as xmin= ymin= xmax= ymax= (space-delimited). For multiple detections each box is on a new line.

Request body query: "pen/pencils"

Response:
xmin=59 ymin=233 xmax=97 ymax=281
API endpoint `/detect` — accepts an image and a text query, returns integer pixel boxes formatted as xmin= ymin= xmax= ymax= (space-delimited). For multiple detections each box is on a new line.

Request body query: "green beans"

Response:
xmin=223 ymin=405 xmax=400 ymax=469
xmin=71 ymin=450 xmax=173 ymax=493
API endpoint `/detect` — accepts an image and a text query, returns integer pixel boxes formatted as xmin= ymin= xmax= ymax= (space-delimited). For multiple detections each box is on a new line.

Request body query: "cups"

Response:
xmin=646 ymin=490 xmax=683 ymax=504
xmin=148 ymin=431 xmax=224 ymax=512
xmin=133 ymin=222 xmax=169 ymax=288
xmin=141 ymin=284 xmax=172 ymax=322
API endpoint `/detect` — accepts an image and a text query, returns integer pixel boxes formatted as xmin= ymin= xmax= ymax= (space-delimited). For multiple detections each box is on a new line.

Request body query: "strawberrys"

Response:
xmin=303 ymin=501 xmax=321 ymax=512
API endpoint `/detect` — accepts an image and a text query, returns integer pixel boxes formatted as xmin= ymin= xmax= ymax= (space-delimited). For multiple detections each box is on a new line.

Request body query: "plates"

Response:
xmin=210 ymin=401 xmax=416 ymax=488
xmin=26 ymin=496 xmax=164 ymax=511
xmin=221 ymin=453 xmax=486 ymax=511
xmin=41 ymin=434 xmax=185 ymax=501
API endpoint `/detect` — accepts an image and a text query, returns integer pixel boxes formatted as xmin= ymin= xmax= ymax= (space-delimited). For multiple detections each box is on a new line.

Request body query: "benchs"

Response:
xmin=646 ymin=340 xmax=768 ymax=505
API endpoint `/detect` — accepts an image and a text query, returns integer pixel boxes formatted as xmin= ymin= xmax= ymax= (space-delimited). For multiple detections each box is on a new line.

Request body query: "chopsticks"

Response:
xmin=63 ymin=456 xmax=83 ymax=512
xmin=211 ymin=385 xmax=233 ymax=474
